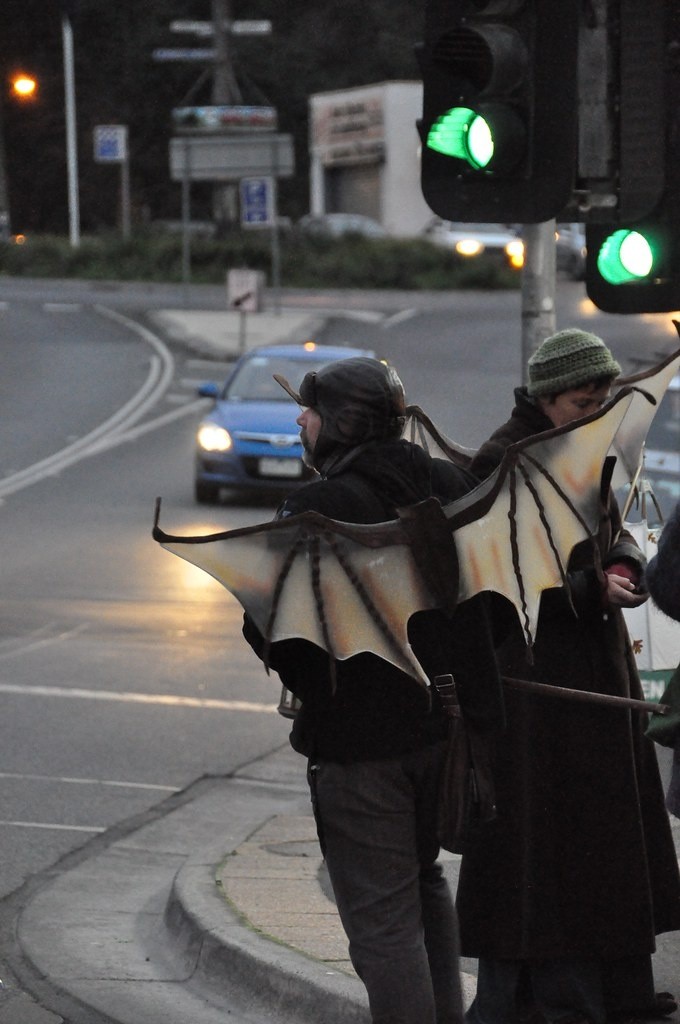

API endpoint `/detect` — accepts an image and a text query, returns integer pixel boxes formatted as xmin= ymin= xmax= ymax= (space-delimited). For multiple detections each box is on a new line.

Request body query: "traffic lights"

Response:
xmin=415 ymin=0 xmax=580 ymax=226
xmin=587 ymin=0 xmax=680 ymax=315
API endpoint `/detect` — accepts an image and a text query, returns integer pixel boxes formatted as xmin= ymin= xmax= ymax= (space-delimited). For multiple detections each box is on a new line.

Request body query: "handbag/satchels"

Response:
xmin=433 ymin=671 xmax=497 ymax=852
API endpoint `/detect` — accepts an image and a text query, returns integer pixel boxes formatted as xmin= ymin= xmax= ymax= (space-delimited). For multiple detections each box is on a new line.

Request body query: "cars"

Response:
xmin=556 ymin=223 xmax=586 ymax=279
xmin=616 ymin=350 xmax=680 ymax=705
xmin=299 ymin=213 xmax=396 ymax=242
xmin=419 ymin=213 xmax=524 ymax=288
xmin=193 ymin=344 xmax=406 ymax=504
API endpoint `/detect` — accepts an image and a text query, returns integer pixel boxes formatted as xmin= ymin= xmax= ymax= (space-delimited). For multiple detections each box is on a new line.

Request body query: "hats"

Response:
xmin=527 ymin=329 xmax=621 ymax=396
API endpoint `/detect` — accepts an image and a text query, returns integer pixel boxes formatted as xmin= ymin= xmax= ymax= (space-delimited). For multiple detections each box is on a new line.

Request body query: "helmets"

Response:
xmin=299 ymin=357 xmax=405 ymax=476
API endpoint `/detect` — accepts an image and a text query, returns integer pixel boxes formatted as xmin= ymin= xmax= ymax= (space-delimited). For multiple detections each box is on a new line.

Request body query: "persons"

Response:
xmin=455 ymin=328 xmax=680 ymax=1024
xmin=243 ymin=358 xmax=488 ymax=1024
